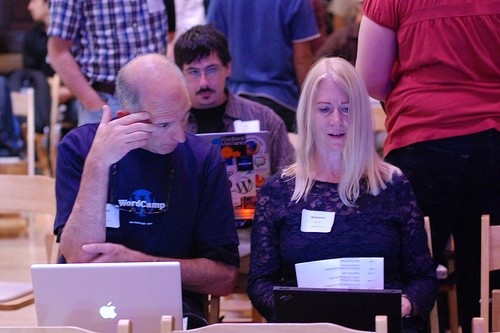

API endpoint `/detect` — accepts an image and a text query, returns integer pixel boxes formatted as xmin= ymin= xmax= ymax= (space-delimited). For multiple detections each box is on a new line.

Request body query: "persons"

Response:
xmin=172 ymin=25 xmax=296 ymax=175
xmin=0 ymin=1 xmax=361 ymax=160
xmin=247 ymin=56 xmax=438 ymax=324
xmin=354 ymin=0 xmax=500 ymax=333
xmin=52 ymin=55 xmax=240 ymax=330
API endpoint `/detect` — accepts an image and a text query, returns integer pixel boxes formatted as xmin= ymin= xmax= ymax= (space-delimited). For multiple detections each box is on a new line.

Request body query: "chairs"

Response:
xmin=0 ymin=53 xmax=500 ymax=333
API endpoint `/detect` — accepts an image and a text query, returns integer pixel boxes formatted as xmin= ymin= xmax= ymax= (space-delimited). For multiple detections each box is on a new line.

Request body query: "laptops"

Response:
xmin=193 ymin=131 xmax=271 ymax=227
xmin=30 ymin=262 xmax=188 ymax=333
xmin=272 ymin=286 xmax=402 ymax=333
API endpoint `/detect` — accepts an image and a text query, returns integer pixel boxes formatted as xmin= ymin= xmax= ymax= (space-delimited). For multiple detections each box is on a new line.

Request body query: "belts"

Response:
xmin=86 ymin=77 xmax=115 ymax=94
